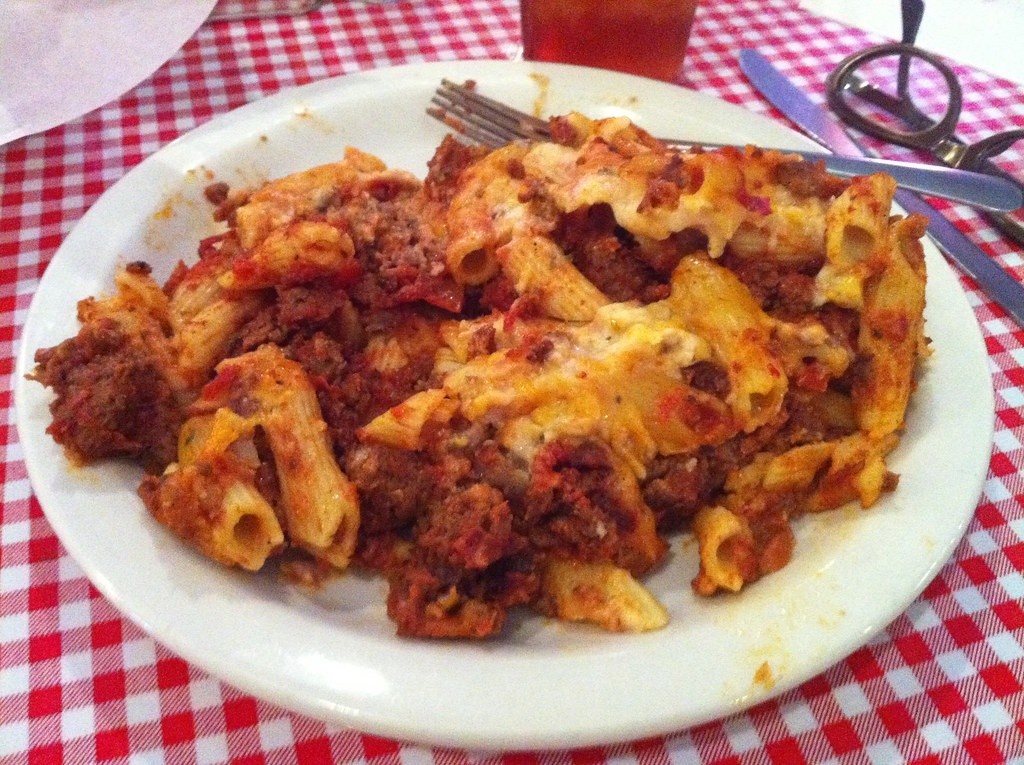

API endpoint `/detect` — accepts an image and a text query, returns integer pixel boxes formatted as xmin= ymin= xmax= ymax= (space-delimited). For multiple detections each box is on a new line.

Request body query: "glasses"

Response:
xmin=826 ymin=0 xmax=1024 ymax=250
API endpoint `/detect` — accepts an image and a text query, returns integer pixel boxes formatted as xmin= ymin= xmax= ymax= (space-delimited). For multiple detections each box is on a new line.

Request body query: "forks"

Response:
xmin=426 ymin=77 xmax=1023 ymax=215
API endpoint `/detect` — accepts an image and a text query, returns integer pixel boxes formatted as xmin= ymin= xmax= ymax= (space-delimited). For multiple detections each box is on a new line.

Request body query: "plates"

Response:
xmin=15 ymin=60 xmax=997 ymax=749
xmin=1 ymin=1 xmax=218 ymax=145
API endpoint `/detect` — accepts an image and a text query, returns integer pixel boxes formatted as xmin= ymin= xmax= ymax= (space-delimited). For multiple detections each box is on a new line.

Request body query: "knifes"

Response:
xmin=740 ymin=45 xmax=1024 ymax=362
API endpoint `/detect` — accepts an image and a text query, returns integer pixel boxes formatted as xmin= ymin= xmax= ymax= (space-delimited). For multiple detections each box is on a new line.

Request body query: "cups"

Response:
xmin=520 ymin=0 xmax=700 ymax=87
